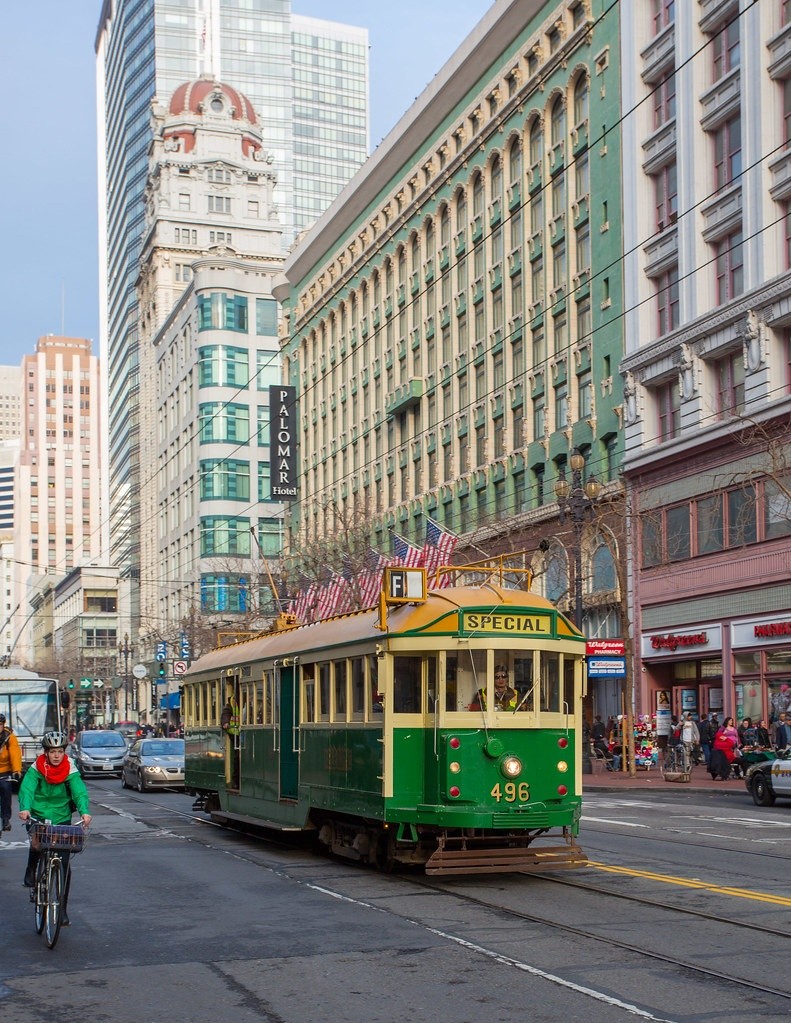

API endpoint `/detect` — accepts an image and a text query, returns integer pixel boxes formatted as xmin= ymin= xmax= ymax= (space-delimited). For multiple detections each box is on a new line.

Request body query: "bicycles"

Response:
xmin=0 ymin=774 xmax=20 ymax=839
xmin=665 ymin=744 xmax=692 ymax=774
xmin=21 ymin=815 xmax=85 ymax=952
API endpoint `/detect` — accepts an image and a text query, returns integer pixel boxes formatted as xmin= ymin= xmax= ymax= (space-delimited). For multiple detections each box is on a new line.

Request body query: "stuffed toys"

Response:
xmin=618 ymin=713 xmax=658 ymax=756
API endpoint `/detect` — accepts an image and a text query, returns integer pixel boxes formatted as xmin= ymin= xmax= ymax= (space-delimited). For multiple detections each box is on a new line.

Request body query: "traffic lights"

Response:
xmin=160 ymin=662 xmax=165 ymax=676
xmin=69 ymin=678 xmax=74 ymax=689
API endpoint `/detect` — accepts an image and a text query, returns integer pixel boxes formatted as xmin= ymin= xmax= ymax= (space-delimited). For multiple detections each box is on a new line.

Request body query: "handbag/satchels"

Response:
xmin=36 ymin=822 xmax=84 ymax=844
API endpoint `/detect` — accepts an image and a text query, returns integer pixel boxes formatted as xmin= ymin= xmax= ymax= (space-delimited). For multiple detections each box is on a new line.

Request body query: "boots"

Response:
xmin=56 ymin=873 xmax=72 ymax=927
xmin=24 ymin=842 xmax=42 ymax=886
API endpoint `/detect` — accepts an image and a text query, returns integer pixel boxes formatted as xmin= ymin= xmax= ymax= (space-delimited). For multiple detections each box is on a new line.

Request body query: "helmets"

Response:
xmin=0 ymin=714 xmax=6 ymax=723
xmin=42 ymin=731 xmax=69 ymax=748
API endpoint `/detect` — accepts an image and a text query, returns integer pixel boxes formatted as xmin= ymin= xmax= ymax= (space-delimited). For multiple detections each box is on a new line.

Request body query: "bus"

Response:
xmin=0 ymin=671 xmax=70 ymax=778
xmin=178 ymin=564 xmax=590 ymax=876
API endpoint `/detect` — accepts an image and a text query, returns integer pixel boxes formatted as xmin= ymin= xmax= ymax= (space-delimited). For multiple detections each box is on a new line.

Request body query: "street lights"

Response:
xmin=556 ymin=446 xmax=600 ymax=632
xmin=119 ymin=632 xmax=135 ymax=721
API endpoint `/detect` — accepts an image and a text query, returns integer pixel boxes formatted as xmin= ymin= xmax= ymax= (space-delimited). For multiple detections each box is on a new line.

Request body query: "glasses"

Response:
xmin=495 ymin=674 xmax=508 ymax=680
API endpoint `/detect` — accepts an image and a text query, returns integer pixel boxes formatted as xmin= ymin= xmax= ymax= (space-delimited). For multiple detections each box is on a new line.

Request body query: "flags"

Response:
xmin=287 ymin=562 xmax=353 ymax=622
xmin=342 ymin=550 xmax=392 ymax=611
xmin=393 ymin=518 xmax=458 ymax=589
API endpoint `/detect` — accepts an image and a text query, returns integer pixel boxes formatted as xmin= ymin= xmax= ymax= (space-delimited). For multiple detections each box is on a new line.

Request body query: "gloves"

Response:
xmin=12 ymin=771 xmax=22 ymax=780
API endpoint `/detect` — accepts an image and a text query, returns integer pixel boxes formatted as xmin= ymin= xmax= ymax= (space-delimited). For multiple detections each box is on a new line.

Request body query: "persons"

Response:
xmin=65 ymin=724 xmax=100 ymax=742
xmin=18 ymin=731 xmax=92 ymax=926
xmin=137 ymin=722 xmax=185 ymax=739
xmin=0 ymin=713 xmax=22 ymax=831
xmin=660 ymin=691 xmax=669 ymax=704
xmin=756 ymin=710 xmax=791 ymax=750
xmin=468 ymin=665 xmax=527 ymax=711
xmin=716 ymin=717 xmax=756 ymax=779
xmin=221 ymin=697 xmax=239 ymax=770
xmin=582 ymin=715 xmax=623 ymax=772
xmin=668 ymin=711 xmax=721 ymax=765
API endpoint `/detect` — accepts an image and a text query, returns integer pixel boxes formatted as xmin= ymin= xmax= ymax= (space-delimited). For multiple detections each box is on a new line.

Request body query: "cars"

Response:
xmin=71 ymin=729 xmax=134 ymax=779
xmin=120 ymin=738 xmax=186 ymax=793
xmin=114 ymin=720 xmax=142 ymax=740
xmin=745 ymin=745 xmax=791 ymax=806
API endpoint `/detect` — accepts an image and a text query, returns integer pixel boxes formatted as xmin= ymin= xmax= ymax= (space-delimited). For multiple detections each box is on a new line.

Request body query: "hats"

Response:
xmin=494 ymin=664 xmax=508 ymax=674
xmin=701 ymin=714 xmax=707 ymax=719
xmin=711 ymin=713 xmax=718 ymax=717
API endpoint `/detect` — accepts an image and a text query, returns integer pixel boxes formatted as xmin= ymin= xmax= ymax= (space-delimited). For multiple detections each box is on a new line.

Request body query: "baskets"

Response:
xmin=27 ymin=823 xmax=85 ymax=852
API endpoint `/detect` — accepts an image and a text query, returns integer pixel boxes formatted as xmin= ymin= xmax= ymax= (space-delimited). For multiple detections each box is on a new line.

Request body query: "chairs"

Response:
xmin=590 ymin=747 xmax=617 ymax=775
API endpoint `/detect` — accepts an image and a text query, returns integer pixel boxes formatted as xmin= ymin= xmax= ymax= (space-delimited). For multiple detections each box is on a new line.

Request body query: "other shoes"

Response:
xmin=232 ymin=783 xmax=239 ymax=789
xmin=3 ymin=820 xmax=12 ymax=831
xmin=609 ymin=767 xmax=613 ymax=770
xmin=615 ymin=768 xmax=620 ymax=771
xmin=695 ymin=760 xmax=700 ymax=766
